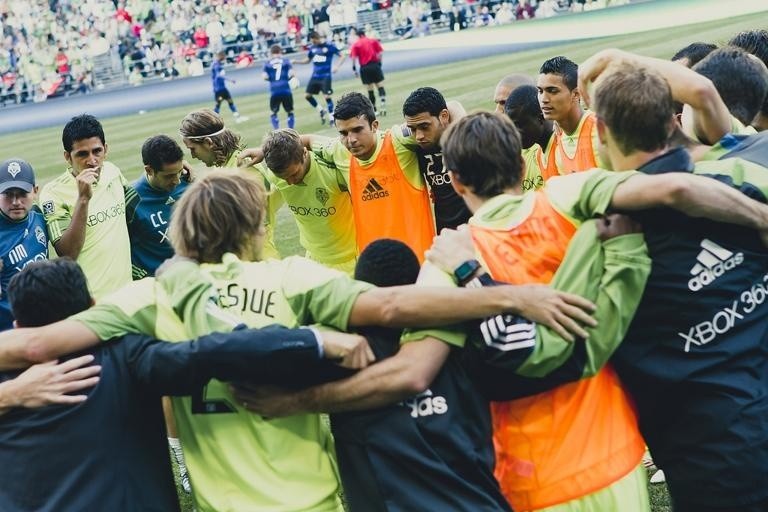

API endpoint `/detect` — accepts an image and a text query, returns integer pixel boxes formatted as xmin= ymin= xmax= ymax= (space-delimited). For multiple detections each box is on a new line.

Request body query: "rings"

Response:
xmin=242 ymin=401 xmax=249 ymax=409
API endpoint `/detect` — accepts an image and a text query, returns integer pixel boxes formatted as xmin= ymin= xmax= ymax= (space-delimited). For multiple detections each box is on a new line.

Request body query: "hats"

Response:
xmin=0 ymin=160 xmax=38 ymax=194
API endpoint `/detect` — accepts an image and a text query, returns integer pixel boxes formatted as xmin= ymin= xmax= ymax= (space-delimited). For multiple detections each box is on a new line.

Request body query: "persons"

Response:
xmin=577 ymin=45 xmax=768 ymax=147
xmin=211 ymin=52 xmax=242 ymax=119
xmin=218 ymin=213 xmax=651 ymax=512
xmin=666 ymin=41 xmax=717 ymax=70
xmin=0 ymin=256 xmax=377 ymax=512
xmin=349 ymin=30 xmax=388 ymax=118
xmin=0 ymin=167 xmax=599 ymax=512
xmin=213 ymin=0 xmax=269 ymax=62
xmin=670 ymin=114 xmax=768 ymax=169
xmin=423 ymin=59 xmax=768 ymax=512
xmin=310 ymin=0 xmax=380 ymax=49
xmin=536 ymin=57 xmax=615 ymax=175
xmin=236 ymin=92 xmax=469 ymax=267
xmin=229 ymin=109 xmax=768 ymax=512
xmin=106 ymin=0 xmax=213 ymax=84
xmin=401 ymin=86 xmax=473 ymax=233
xmin=0 ymin=354 xmax=102 ymax=418
xmin=728 ymin=29 xmax=767 ymax=133
xmin=381 ymin=0 xmax=598 ymax=40
xmin=256 ymin=128 xmax=359 ymax=279
xmin=261 ymin=45 xmax=297 ymax=129
xmin=290 ymin=33 xmax=345 ymax=126
xmin=179 ymin=108 xmax=287 ymax=261
xmin=1 ymin=159 xmax=51 ymax=331
xmin=493 ymin=72 xmax=543 ymax=192
xmin=2 ymin=1 xmax=106 ymax=109
xmin=270 ymin=0 xmax=310 ymax=56
xmin=124 ymin=135 xmax=198 ymax=281
xmin=503 ymin=84 xmax=566 ymax=183
xmin=36 ymin=114 xmax=140 ymax=308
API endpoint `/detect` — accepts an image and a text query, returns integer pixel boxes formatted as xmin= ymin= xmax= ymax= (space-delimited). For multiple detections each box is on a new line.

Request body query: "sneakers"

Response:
xmin=380 ymin=107 xmax=386 ymax=117
xmin=375 ymin=111 xmax=379 ymax=116
xmin=321 ymin=111 xmax=327 ymax=125
xmin=329 ymin=113 xmax=334 ymax=127
xmin=235 ymin=116 xmax=248 ymax=124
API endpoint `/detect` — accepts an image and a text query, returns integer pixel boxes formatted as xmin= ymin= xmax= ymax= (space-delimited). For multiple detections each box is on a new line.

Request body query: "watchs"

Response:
xmin=453 ymin=259 xmax=481 ymax=288
